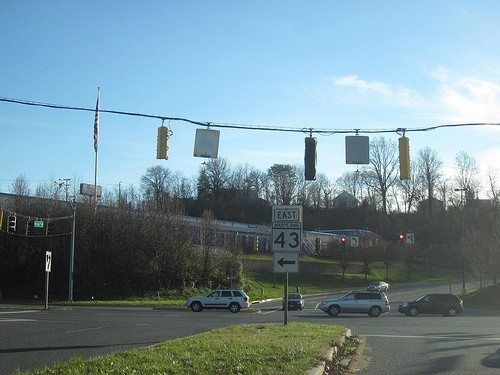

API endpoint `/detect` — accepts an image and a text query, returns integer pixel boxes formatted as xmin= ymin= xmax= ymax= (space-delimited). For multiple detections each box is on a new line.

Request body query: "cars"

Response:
xmin=281 ymin=293 xmax=305 ymax=311
xmin=368 ymin=281 xmax=388 ymax=292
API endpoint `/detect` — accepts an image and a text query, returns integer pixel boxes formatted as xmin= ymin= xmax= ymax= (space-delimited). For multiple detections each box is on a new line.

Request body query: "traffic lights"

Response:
xmin=399 ymin=233 xmax=404 ymax=244
xmin=25 ymin=219 xmax=34 ymax=237
xmin=7 ymin=214 xmax=17 ymax=235
xmin=341 ymin=237 xmax=346 ymax=247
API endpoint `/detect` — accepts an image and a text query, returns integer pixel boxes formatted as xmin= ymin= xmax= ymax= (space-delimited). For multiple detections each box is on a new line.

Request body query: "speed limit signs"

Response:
xmin=271 ymin=221 xmax=303 ymax=251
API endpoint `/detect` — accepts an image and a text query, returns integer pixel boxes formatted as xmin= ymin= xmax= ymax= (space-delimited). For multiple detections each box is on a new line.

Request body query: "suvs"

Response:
xmin=397 ymin=293 xmax=465 ymax=318
xmin=319 ymin=289 xmax=391 ymax=317
xmin=186 ymin=289 xmax=251 ymax=313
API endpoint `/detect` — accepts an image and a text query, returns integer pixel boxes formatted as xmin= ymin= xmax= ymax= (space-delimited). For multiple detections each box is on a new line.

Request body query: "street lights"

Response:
xmin=455 ymin=188 xmax=469 ymax=290
xmin=62 ymin=178 xmax=71 ymax=202
